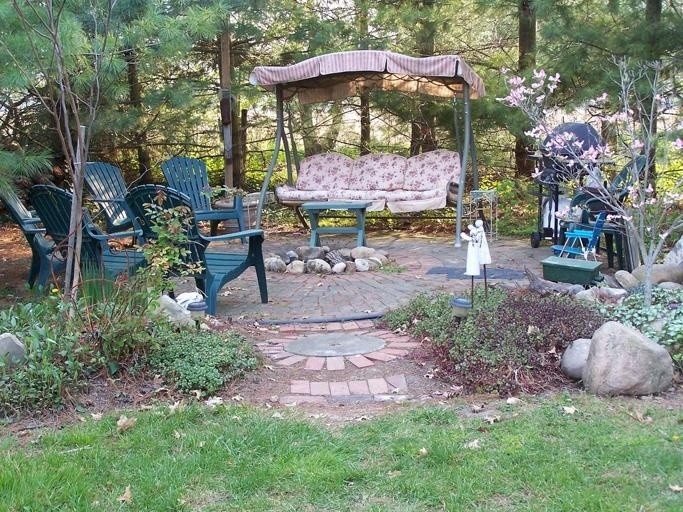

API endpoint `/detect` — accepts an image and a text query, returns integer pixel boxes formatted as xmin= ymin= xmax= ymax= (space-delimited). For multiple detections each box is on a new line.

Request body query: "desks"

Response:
xmin=301 ymin=202 xmax=370 ymax=249
xmin=470 ymin=189 xmax=496 ymax=247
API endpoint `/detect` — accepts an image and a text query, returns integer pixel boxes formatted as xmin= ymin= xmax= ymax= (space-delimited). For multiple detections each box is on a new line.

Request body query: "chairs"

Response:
xmin=552 ymin=157 xmax=646 ymax=274
